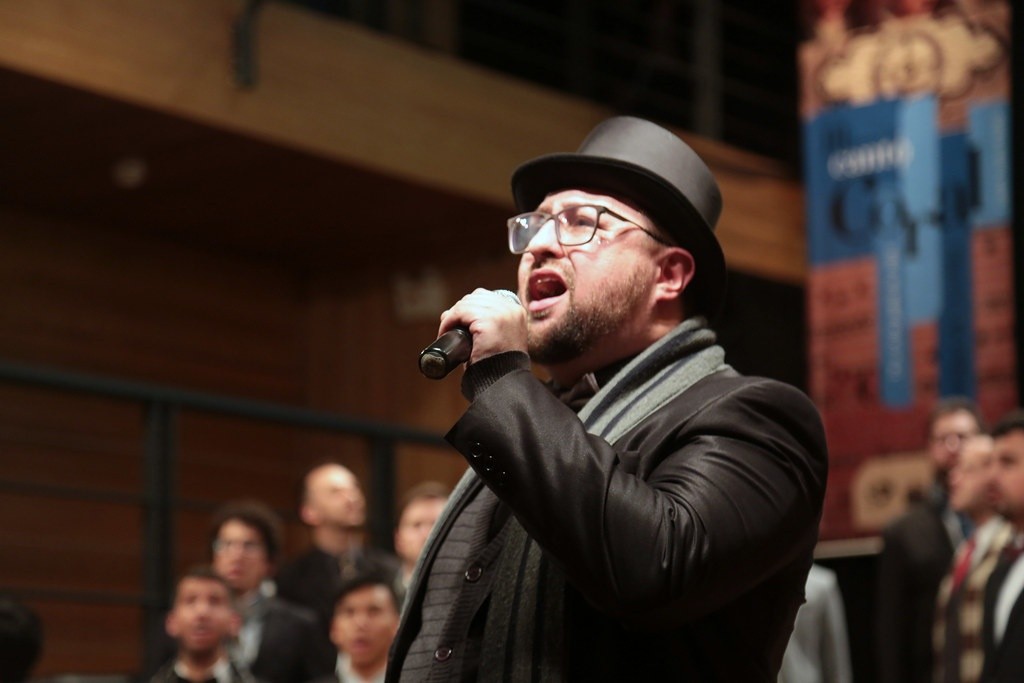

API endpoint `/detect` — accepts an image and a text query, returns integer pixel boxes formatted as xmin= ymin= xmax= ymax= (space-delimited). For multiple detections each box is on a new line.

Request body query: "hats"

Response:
xmin=511 ymin=116 xmax=728 ymax=308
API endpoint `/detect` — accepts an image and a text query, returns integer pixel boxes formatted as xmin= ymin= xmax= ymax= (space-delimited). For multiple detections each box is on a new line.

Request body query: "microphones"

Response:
xmin=419 ymin=289 xmax=521 ymax=379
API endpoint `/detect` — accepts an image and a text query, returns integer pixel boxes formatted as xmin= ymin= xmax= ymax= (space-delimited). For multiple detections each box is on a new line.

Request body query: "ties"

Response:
xmin=952 ymin=538 xmax=975 ymax=593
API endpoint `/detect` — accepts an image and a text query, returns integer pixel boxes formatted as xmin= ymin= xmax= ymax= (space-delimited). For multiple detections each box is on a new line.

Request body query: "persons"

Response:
xmin=777 ymin=393 xmax=1024 ymax=683
xmin=382 ymin=115 xmax=829 ymax=683
xmin=152 ymin=459 xmax=447 ymax=683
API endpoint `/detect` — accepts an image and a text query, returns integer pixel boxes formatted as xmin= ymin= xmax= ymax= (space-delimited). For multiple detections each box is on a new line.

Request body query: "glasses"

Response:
xmin=507 ymin=205 xmax=669 ymax=254
